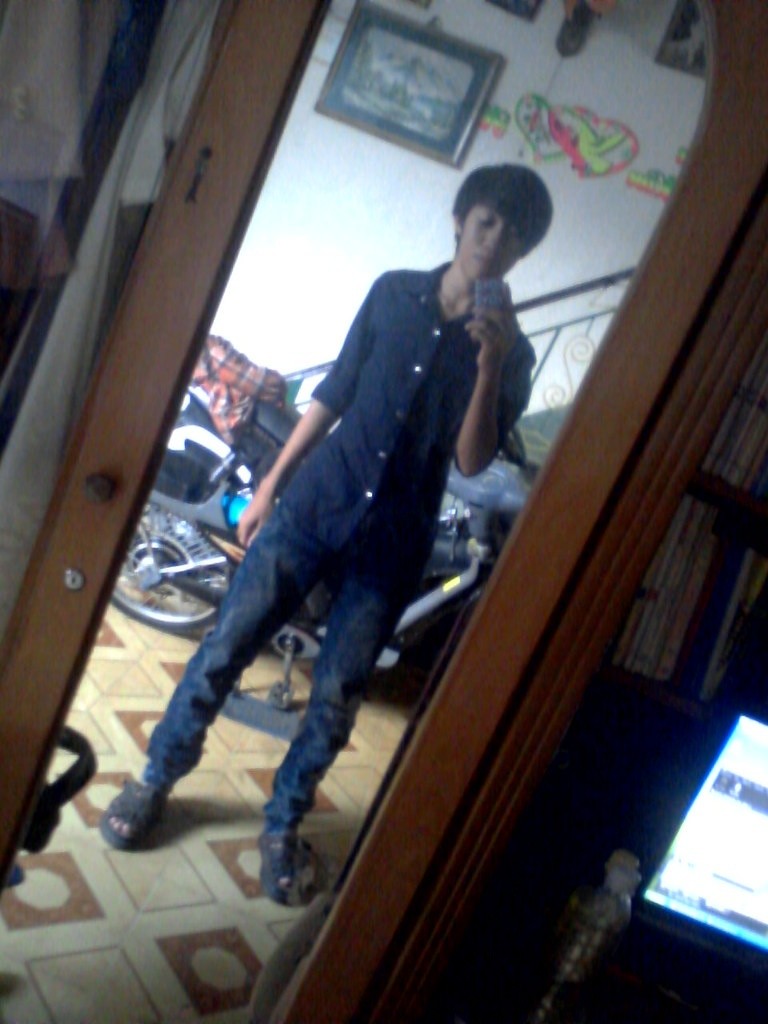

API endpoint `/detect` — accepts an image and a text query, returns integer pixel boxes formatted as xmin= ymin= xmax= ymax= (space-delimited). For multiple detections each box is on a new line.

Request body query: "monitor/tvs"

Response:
xmin=631 ymin=711 xmax=768 ymax=975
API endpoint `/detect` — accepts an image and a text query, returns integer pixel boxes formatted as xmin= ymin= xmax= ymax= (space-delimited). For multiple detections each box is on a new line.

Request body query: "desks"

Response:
xmin=525 ymin=848 xmax=768 ymax=1024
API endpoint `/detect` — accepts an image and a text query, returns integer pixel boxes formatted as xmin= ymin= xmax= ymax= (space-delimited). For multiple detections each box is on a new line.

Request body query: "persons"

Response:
xmin=101 ymin=165 xmax=552 ymax=906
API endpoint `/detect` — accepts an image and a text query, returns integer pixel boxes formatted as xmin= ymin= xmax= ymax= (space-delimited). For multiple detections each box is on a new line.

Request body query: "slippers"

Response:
xmin=100 ymin=776 xmax=168 ymax=849
xmin=258 ymin=826 xmax=305 ymax=904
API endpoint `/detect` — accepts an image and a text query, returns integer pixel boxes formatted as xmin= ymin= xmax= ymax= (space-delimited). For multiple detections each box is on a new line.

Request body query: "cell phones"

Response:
xmin=474 ymin=277 xmax=507 ymax=333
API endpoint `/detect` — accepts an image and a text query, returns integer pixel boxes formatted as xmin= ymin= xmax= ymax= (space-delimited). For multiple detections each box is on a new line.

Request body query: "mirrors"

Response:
xmin=0 ymin=0 xmax=768 ymax=1024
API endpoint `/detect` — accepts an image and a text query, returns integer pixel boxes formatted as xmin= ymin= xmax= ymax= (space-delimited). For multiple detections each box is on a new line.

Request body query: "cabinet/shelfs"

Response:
xmin=602 ymin=476 xmax=768 ymax=726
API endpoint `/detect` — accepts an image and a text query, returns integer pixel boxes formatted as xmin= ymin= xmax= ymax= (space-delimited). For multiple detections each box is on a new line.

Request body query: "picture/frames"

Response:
xmin=314 ymin=3 xmax=509 ymax=171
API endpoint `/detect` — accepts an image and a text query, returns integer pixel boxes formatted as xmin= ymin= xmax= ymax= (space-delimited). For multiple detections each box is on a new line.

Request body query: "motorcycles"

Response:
xmin=105 ymin=413 xmax=529 ymax=709
